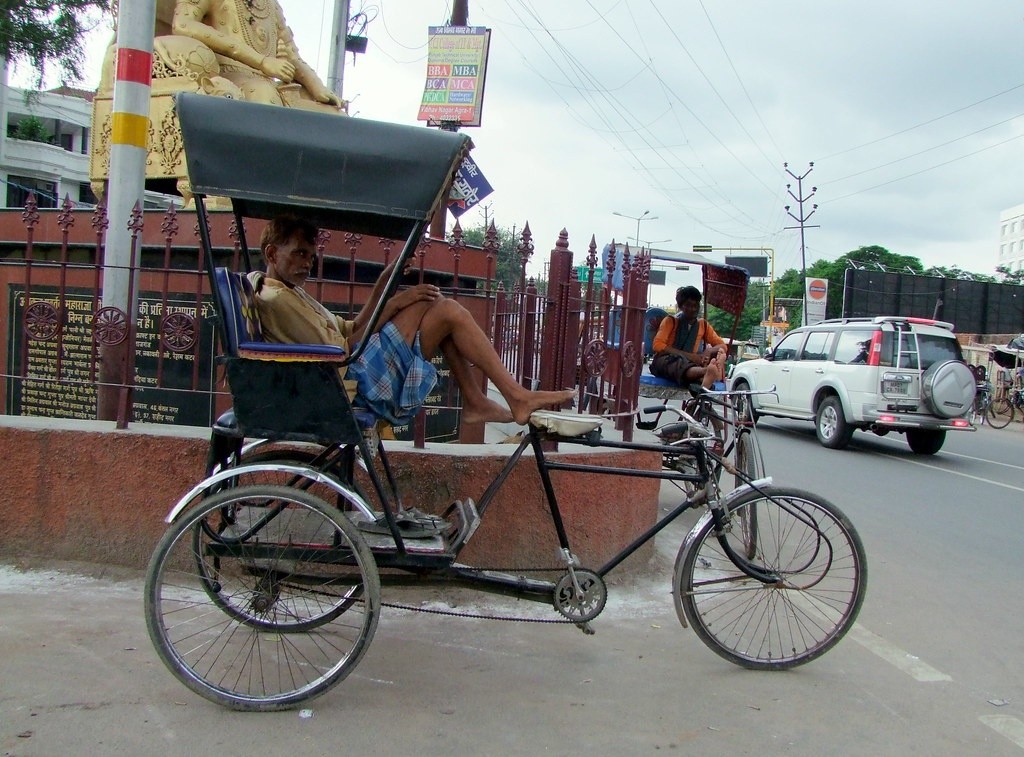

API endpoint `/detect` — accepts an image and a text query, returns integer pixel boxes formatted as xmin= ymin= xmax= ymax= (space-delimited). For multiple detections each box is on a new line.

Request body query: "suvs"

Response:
xmin=726 ymin=315 xmax=977 ymax=454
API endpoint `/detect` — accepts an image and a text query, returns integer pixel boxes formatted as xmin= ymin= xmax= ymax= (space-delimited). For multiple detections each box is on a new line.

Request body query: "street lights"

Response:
xmin=612 ymin=210 xmax=672 ymax=249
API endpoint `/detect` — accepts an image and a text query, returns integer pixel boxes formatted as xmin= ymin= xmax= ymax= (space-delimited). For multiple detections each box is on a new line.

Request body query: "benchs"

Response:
xmin=209 ymin=271 xmax=384 ymax=449
xmin=607 ymin=306 xmax=727 ymax=402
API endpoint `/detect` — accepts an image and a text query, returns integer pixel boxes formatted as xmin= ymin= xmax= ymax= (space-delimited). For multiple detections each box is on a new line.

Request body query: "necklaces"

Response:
xmin=234 ymin=0 xmax=275 ymax=57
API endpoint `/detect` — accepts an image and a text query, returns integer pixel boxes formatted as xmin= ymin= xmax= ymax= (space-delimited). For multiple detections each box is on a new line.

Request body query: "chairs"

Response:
xmin=91 ymin=0 xmax=303 ymax=211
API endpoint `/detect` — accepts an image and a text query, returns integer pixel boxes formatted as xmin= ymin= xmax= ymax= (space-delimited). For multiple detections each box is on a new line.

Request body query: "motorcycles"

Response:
xmin=720 ymin=337 xmax=760 ymax=381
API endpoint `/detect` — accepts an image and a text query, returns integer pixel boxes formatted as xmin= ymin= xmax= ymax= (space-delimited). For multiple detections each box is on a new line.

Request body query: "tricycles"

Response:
xmin=141 ymin=90 xmax=866 ymax=713
xmin=968 ymin=381 xmax=992 ymax=426
xmin=597 ymin=242 xmax=781 ymax=562
xmin=985 ymin=336 xmax=1024 ymax=430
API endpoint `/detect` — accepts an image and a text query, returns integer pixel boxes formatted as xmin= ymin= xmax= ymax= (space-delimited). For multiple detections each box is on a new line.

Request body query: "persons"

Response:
xmin=648 ymin=286 xmax=727 ymax=390
xmin=172 ymin=0 xmax=342 ymax=108
xmin=246 ymin=215 xmax=578 ymax=426
